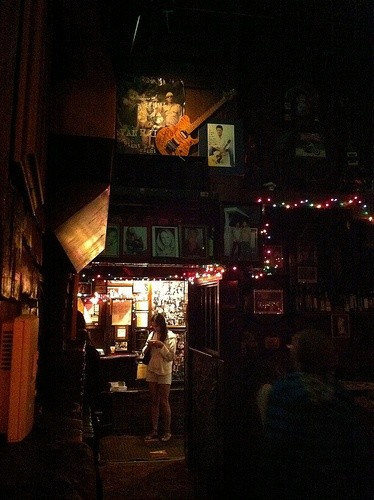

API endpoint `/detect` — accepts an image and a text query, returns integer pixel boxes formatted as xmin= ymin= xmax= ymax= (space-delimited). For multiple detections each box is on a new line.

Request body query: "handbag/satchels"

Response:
xmin=139 ymin=351 xmax=151 ymax=365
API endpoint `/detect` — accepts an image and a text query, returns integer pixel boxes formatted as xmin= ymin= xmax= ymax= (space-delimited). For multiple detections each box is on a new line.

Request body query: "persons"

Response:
xmin=209 ymin=124 xmax=235 ymax=168
xmin=77 ymin=310 xmax=92 ymax=345
xmin=338 ymin=317 xmax=345 ymax=336
xmin=161 ymin=92 xmax=185 ymax=127
xmin=84 ymin=349 xmax=111 ymax=435
xmin=264 ymin=329 xmax=374 ymax=500
xmin=230 ymin=221 xmax=252 ymax=260
xmin=143 ymin=312 xmax=176 ymax=441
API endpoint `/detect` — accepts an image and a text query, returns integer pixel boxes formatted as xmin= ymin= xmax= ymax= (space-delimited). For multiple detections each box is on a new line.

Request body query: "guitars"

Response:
xmin=209 ymin=139 xmax=231 ymax=165
xmin=154 ymin=88 xmax=238 ymax=156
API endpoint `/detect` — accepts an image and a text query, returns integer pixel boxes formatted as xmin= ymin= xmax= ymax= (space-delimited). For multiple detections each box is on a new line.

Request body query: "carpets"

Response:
xmin=100 ymin=437 xmax=171 ymax=460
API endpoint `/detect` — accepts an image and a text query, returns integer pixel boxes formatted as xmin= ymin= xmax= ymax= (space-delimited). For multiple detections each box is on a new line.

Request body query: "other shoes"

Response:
xmin=144 ymin=430 xmax=159 ymax=442
xmin=160 ymin=430 xmax=172 ymax=442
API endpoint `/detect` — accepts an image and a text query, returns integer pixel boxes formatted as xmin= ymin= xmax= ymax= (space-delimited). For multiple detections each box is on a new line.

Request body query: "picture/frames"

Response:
xmin=253 ymin=289 xmax=284 ymax=314
xmin=197 ymin=121 xmax=245 ymax=175
xmin=178 ymin=224 xmax=208 ymax=258
xmin=151 ymin=226 xmax=179 ymax=258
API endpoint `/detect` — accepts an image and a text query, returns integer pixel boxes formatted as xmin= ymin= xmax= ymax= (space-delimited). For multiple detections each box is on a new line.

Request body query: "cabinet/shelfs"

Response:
xmin=302 ymin=310 xmax=374 ymax=369
